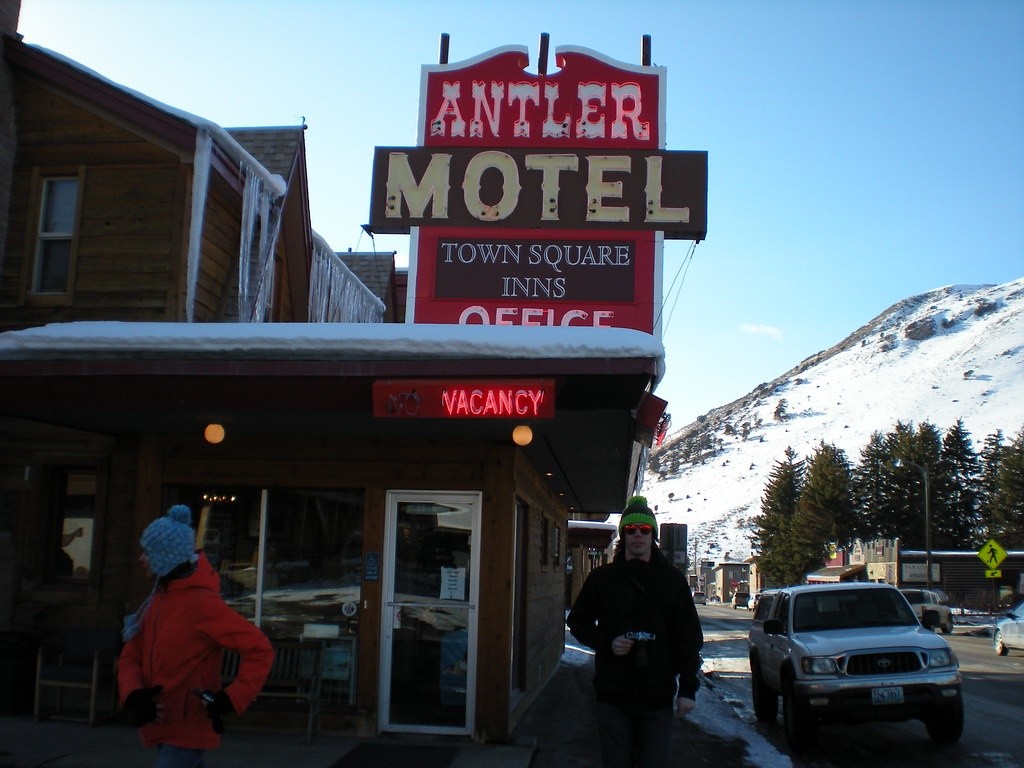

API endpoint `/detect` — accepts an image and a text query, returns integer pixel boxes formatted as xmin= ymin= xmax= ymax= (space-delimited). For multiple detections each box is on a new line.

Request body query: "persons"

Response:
xmin=566 ymin=497 xmax=703 ymax=768
xmin=117 ymin=505 xmax=276 ymax=768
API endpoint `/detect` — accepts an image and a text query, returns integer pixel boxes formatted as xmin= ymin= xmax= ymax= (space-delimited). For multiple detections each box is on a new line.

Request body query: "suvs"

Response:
xmin=732 ymin=591 xmax=751 ymax=610
xmin=748 ymin=592 xmax=763 ymax=612
xmin=749 ymin=584 xmax=965 ymax=755
xmin=691 ymin=591 xmax=708 ymax=606
xmin=899 ymin=588 xmax=954 ymax=635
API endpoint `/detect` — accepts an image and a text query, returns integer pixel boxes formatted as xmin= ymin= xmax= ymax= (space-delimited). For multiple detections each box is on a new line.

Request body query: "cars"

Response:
xmin=992 ymin=600 xmax=1024 ymax=656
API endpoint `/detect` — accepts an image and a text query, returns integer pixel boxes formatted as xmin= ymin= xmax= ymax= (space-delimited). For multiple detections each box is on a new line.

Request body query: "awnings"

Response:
xmin=0 ymin=322 xmax=655 ymax=440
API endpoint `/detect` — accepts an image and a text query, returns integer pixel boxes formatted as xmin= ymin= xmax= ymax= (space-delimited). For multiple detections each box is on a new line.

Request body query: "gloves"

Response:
xmin=123 ymin=685 xmax=165 ymax=728
xmin=200 ymin=689 xmax=234 ymax=733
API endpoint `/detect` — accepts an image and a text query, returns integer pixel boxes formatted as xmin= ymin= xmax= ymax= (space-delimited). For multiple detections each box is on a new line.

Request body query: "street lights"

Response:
xmin=895 ymin=459 xmax=932 ymax=589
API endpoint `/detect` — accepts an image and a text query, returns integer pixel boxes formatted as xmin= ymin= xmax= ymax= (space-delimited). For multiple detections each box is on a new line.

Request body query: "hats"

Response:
xmin=121 ymin=505 xmax=196 ymax=642
xmin=619 ymin=495 xmax=658 ymax=540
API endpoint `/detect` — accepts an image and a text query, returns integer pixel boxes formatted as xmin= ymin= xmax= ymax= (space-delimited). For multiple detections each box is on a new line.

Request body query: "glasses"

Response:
xmin=623 ymin=525 xmax=652 ymax=535
